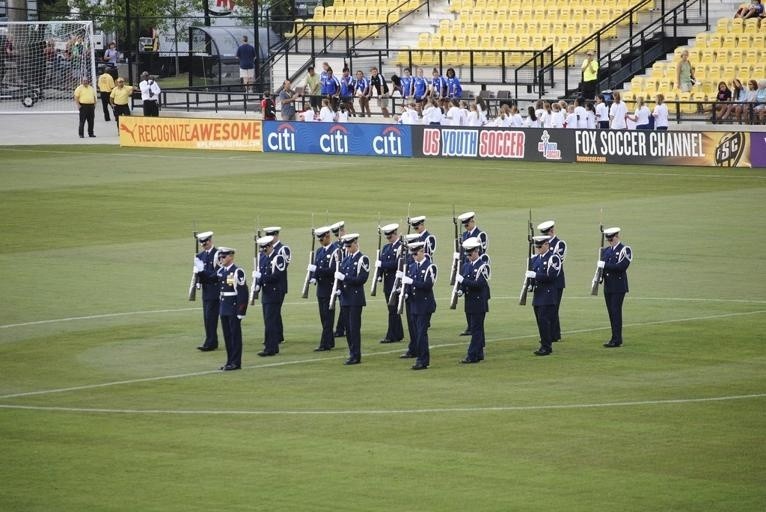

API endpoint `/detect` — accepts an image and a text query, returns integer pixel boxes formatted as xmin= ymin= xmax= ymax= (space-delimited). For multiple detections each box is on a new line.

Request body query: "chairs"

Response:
xmin=392 ymin=0 xmax=657 ymax=70
xmin=619 ymin=2 xmax=766 ymax=114
xmin=284 ymin=0 xmax=419 ymax=40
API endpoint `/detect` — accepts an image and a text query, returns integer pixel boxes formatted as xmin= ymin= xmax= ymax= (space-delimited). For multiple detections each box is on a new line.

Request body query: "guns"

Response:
xmin=517 ymin=207 xmax=535 ymax=305
xmin=591 ymin=207 xmax=604 ymax=296
xmin=189 ymin=201 xmax=464 ymax=316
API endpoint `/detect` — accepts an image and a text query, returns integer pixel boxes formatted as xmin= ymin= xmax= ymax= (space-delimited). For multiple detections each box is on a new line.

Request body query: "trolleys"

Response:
xmin=0 ymin=62 xmax=42 ymax=109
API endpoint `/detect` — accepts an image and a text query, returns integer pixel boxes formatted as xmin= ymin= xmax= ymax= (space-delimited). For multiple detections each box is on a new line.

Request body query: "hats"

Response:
xmin=462 ymin=237 xmax=481 ymax=254
xmin=257 ymin=224 xmax=283 ymax=253
xmin=457 ymin=212 xmax=475 ymax=221
xmin=314 ymin=220 xmax=361 ymax=246
xmin=603 ymin=227 xmax=620 ymax=238
xmin=380 ymin=215 xmax=428 ymax=252
xmin=532 ymin=220 xmax=556 ymax=248
xmin=196 ymin=231 xmax=237 ymax=260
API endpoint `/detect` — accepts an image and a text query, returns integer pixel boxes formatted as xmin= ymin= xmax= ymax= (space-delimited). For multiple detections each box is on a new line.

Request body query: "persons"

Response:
xmin=0 ymin=27 xmax=161 ymax=139
xmin=261 ymin=91 xmax=276 ymax=121
xmin=735 ymin=0 xmax=766 ymax=20
xmin=279 ymin=80 xmax=298 ymax=121
xmin=236 ymin=35 xmax=257 ymax=94
xmin=597 ymin=227 xmax=633 ymax=347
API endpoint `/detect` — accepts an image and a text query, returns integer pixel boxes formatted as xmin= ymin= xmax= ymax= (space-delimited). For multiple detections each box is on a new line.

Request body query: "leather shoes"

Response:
xmin=313 ymin=329 xmax=349 ymax=352
xmin=257 ymin=340 xmax=286 ymax=356
xmin=198 ymin=344 xmax=218 ymax=351
xmin=460 ymin=329 xmax=484 ymax=364
xmin=218 ymin=362 xmax=241 ymax=370
xmin=603 ymin=340 xmax=622 ymax=348
xmin=380 ymin=337 xmax=430 ymax=371
xmin=533 ymin=347 xmax=553 ymax=356
xmin=343 ymin=358 xmax=361 ymax=365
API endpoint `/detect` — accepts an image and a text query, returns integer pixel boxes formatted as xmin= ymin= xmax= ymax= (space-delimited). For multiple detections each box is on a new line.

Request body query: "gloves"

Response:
xmin=525 ymin=270 xmax=536 ymax=279
xmin=306 ymin=263 xmax=318 ymax=285
xmin=236 ymin=315 xmax=245 ymax=320
xmin=597 ymin=261 xmax=605 ymax=269
xmin=251 ymin=269 xmax=263 ymax=295
xmin=334 ymin=271 xmax=345 ymax=296
xmin=453 ymin=252 xmax=464 ymax=298
xmin=374 ymin=260 xmax=413 ymax=285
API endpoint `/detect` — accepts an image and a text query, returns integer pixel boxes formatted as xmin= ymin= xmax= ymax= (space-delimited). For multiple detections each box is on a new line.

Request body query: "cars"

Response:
xmin=138 ymin=36 xmax=157 ymax=52
